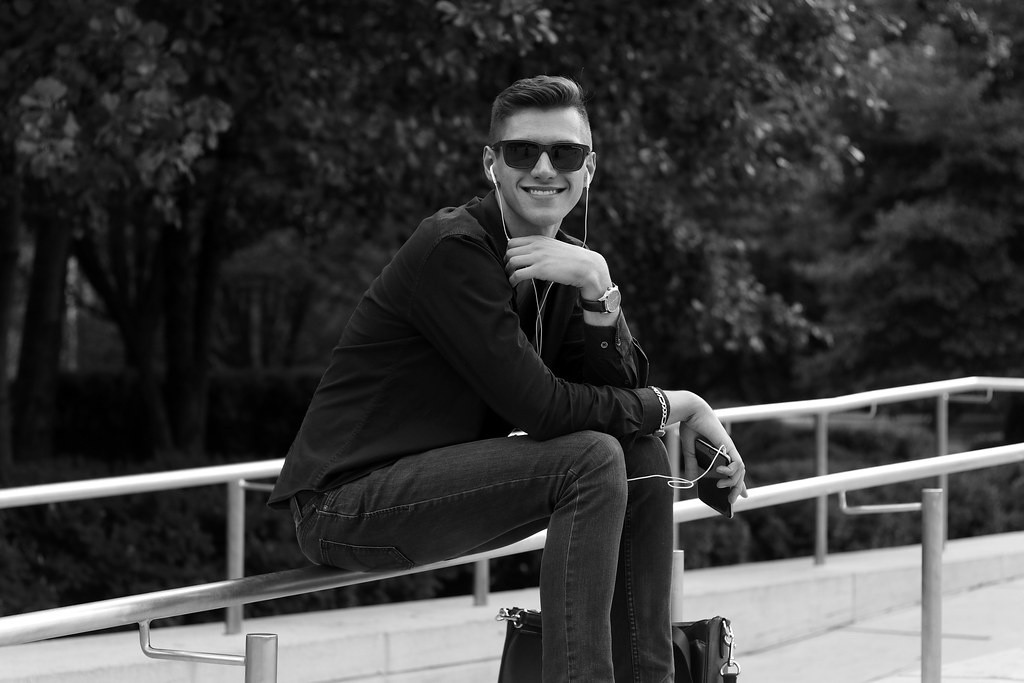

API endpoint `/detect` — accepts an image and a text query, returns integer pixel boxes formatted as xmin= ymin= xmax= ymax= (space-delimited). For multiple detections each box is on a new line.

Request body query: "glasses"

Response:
xmin=491 ymin=139 xmax=592 ymax=172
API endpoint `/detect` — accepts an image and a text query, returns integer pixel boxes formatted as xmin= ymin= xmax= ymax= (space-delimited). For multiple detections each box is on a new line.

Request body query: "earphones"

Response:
xmin=490 ymin=164 xmax=497 ymax=184
xmin=587 ymin=168 xmax=591 ymax=187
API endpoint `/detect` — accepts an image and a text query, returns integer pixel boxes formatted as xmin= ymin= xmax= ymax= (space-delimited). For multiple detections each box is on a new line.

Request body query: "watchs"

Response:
xmin=579 ymin=281 xmax=621 ymax=314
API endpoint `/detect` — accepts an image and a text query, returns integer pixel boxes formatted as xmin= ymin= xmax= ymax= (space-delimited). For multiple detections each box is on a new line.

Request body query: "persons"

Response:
xmin=268 ymin=76 xmax=746 ymax=683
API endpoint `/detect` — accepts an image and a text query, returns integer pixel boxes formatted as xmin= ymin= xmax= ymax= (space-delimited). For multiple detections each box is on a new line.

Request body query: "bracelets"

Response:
xmin=646 ymin=384 xmax=667 ymax=438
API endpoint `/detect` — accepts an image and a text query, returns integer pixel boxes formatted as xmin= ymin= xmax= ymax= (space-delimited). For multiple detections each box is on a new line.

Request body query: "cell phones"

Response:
xmin=695 ymin=439 xmax=733 ymax=519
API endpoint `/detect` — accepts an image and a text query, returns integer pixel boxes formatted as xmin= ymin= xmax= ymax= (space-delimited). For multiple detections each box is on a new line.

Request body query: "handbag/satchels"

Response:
xmin=497 ymin=604 xmax=741 ymax=683
